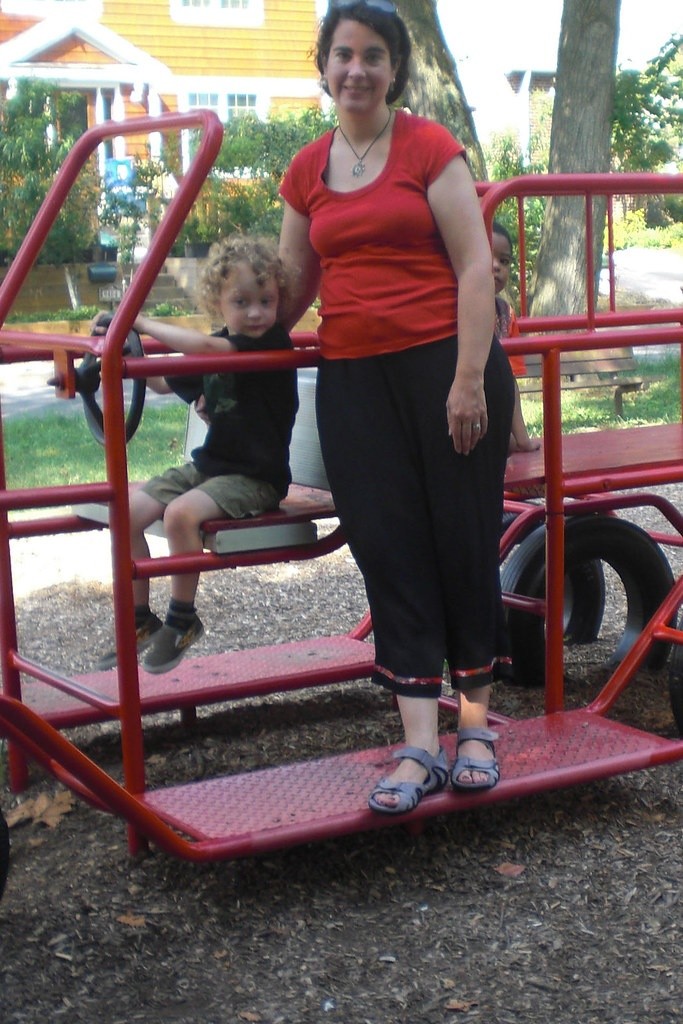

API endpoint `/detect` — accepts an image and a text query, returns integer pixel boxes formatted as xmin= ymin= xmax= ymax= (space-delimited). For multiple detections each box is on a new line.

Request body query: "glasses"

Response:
xmin=327 ymin=0 xmax=399 ymax=17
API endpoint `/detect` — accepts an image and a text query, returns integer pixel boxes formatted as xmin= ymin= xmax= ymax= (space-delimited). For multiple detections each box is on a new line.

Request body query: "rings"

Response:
xmin=472 ymin=423 xmax=481 ymax=434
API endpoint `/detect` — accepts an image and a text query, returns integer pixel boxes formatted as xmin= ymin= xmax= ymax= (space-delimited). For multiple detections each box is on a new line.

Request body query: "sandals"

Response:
xmin=450 ymin=727 xmax=500 ymax=791
xmin=369 ymin=745 xmax=449 ymax=814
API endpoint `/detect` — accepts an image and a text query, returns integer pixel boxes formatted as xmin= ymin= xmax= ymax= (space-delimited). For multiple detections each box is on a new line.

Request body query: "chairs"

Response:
xmin=72 ymin=365 xmax=333 ymax=555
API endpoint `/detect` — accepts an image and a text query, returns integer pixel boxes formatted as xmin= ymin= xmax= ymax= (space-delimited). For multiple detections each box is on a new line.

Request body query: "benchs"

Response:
xmin=515 ymin=346 xmax=664 ymax=421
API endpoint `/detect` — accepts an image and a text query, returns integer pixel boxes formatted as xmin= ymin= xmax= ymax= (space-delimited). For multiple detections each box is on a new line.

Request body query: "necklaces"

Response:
xmin=339 ymin=109 xmax=391 ymax=176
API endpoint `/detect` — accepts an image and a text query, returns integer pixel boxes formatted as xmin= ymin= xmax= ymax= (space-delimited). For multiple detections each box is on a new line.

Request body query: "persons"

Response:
xmin=267 ymin=0 xmax=518 ymax=814
xmin=89 ymin=231 xmax=300 ymax=675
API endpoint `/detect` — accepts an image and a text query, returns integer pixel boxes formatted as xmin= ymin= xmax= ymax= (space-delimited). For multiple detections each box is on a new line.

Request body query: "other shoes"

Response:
xmin=143 ymin=614 xmax=204 ymax=674
xmin=96 ymin=613 xmax=163 ymax=671
